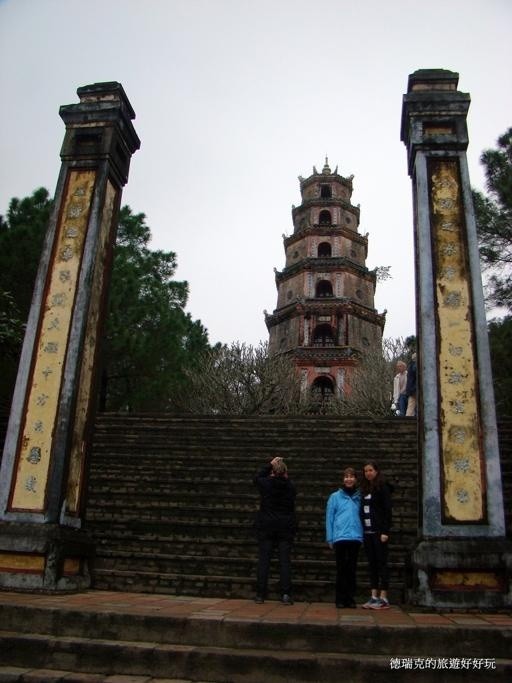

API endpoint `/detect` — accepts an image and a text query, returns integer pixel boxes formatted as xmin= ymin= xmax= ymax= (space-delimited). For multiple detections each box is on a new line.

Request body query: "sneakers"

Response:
xmin=336 ymin=602 xmax=357 ymax=609
xmin=255 ymin=596 xmax=264 ymax=604
xmin=369 ymin=598 xmax=390 ymax=610
xmin=282 ymin=594 xmax=294 ymax=605
xmin=362 ymin=598 xmax=377 ymax=609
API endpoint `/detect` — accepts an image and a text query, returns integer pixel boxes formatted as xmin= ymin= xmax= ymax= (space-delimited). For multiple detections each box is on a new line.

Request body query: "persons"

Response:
xmin=403 ymin=353 xmax=419 ymax=417
xmin=360 ymin=461 xmax=396 ymax=609
xmin=324 ymin=468 xmax=365 ymax=611
xmin=250 ymin=454 xmax=298 ymax=606
xmin=390 ymin=360 xmax=409 ymax=417
